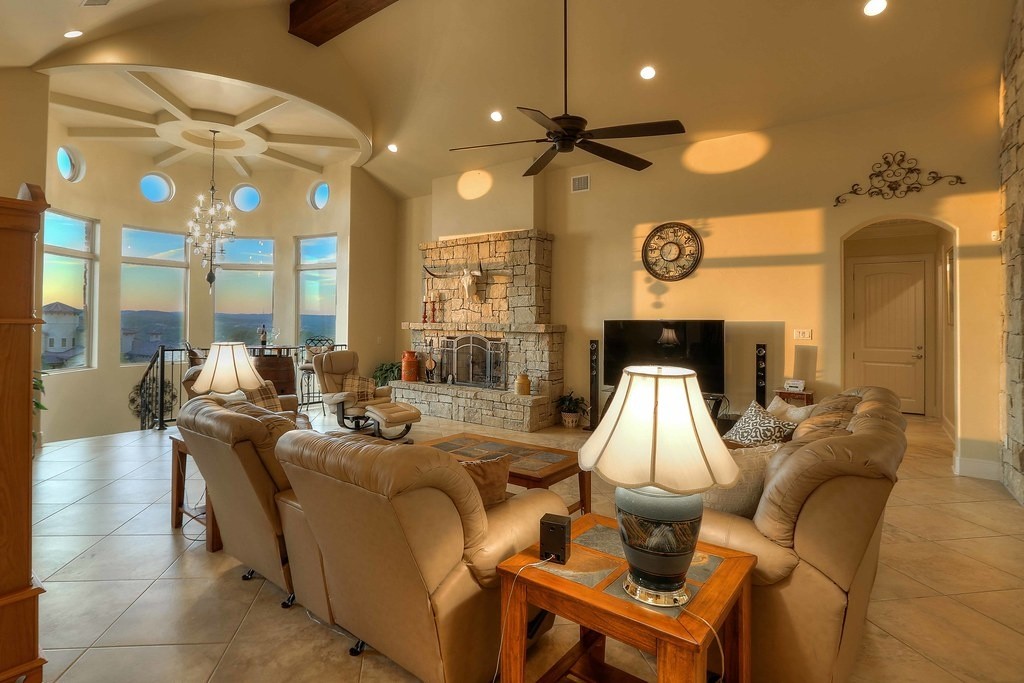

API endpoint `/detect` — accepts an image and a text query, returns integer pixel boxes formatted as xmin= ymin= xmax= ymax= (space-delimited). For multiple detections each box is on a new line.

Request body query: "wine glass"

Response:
xmin=256 ymin=327 xmax=280 ymax=346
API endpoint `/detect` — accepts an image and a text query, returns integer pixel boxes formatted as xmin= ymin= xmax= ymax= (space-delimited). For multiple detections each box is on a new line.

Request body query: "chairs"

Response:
xmin=183 ymin=341 xmax=202 ymax=370
xmin=298 ymin=336 xmax=334 ymax=416
xmin=312 ymin=351 xmax=421 ymax=440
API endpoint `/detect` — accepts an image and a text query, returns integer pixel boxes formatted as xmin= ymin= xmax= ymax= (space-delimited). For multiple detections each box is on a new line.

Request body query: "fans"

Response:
xmin=448 ymin=0 xmax=685 ymax=178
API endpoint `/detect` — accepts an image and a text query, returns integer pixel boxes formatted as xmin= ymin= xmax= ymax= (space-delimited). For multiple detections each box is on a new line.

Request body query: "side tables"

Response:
xmin=496 ymin=513 xmax=758 ymax=683
xmin=168 ymin=433 xmax=223 ymax=552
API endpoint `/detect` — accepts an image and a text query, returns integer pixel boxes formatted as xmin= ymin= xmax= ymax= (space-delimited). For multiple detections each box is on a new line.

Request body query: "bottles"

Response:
xmin=402 ymin=351 xmax=419 ymax=381
xmin=515 ymin=374 xmax=530 ymax=394
xmin=260 ymin=324 xmax=266 ymax=345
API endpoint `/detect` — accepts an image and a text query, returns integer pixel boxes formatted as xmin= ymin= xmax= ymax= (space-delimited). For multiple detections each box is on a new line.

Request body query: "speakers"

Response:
xmin=755 ymin=344 xmax=766 ymax=409
xmin=583 ymin=340 xmax=599 ymax=430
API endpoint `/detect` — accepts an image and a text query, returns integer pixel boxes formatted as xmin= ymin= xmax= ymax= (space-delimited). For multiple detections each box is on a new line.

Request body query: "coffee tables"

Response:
xmin=416 ymin=432 xmax=592 ymax=516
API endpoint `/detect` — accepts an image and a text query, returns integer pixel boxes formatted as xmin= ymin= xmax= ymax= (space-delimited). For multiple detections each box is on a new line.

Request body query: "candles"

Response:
xmin=423 ymin=295 xmax=428 ymax=302
xmin=431 ymin=292 xmax=436 ymax=301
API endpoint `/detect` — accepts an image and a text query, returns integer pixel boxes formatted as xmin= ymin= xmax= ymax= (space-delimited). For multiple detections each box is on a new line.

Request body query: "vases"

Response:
xmin=402 ymin=350 xmax=419 ymax=382
xmin=515 ymin=374 xmax=531 ymax=395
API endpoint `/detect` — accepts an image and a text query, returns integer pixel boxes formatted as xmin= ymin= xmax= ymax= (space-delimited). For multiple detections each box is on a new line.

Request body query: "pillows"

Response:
xmin=702 ymin=442 xmax=787 ymax=518
xmin=721 ymin=399 xmax=797 ymax=447
xmin=342 ymin=373 xmax=375 ymax=402
xmin=240 ymin=380 xmax=282 ymax=412
xmin=459 ymin=452 xmax=511 ymax=510
xmin=305 ymin=345 xmax=334 ymax=363
xmin=766 ymin=395 xmax=818 ymax=424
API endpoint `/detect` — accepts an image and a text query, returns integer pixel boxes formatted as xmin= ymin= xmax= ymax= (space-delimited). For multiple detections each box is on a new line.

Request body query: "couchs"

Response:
xmin=697 ymin=386 xmax=907 ymax=683
xmin=272 ymin=427 xmax=569 ymax=683
xmin=182 ymin=363 xmax=312 ymax=429
xmin=176 ymin=393 xmax=301 ymax=609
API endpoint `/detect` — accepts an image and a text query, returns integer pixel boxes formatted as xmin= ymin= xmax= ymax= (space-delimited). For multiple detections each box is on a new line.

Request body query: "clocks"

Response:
xmin=641 ymin=222 xmax=704 ymax=281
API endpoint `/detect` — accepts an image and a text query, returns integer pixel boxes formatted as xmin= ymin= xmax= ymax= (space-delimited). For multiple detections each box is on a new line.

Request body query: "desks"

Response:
xmin=602 ymin=386 xmax=724 ymax=421
xmin=245 ymin=345 xmax=301 ymax=396
xmin=772 ymin=387 xmax=815 ymax=407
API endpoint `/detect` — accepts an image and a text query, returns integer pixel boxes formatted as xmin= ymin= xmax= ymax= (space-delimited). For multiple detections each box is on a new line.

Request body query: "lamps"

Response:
xmin=578 ymin=365 xmax=740 ymax=607
xmin=657 ymin=328 xmax=680 ymax=361
xmin=187 ymin=129 xmax=237 ymax=295
xmin=191 ymin=342 xmax=266 ymax=403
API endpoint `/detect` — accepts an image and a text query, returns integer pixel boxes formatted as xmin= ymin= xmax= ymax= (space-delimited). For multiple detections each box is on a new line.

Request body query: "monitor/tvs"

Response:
xmin=604 ymin=320 xmax=725 ymax=395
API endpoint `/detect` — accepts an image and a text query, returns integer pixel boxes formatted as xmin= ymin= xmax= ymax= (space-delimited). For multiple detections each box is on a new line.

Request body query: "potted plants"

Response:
xmin=552 ymin=390 xmax=589 ymax=428
